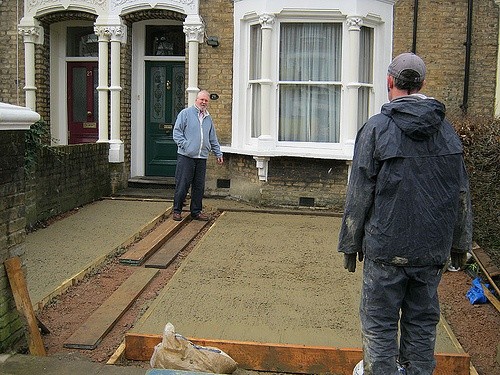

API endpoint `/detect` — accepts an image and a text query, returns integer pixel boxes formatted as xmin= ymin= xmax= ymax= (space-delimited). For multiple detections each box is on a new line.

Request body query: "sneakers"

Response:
xmin=172 ymin=212 xmax=182 ymax=221
xmin=193 ymin=213 xmax=209 ymax=221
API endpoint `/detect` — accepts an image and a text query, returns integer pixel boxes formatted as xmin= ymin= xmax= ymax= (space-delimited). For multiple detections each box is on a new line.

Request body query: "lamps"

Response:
xmin=206 ymin=36 xmax=219 ymax=48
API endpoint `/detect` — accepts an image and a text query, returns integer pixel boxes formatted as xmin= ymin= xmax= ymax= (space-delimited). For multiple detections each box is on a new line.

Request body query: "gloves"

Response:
xmin=450 ymin=249 xmax=467 ymax=270
xmin=343 ymin=251 xmax=364 ymax=272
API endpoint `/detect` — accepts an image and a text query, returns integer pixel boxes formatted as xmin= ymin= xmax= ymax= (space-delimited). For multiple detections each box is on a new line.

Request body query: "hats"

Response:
xmin=388 ymin=52 xmax=426 ymax=82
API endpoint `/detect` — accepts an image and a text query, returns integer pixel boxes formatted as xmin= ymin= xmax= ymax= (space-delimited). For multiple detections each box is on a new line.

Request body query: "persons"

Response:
xmin=337 ymin=52 xmax=474 ymax=375
xmin=172 ymin=90 xmax=223 ymax=221
